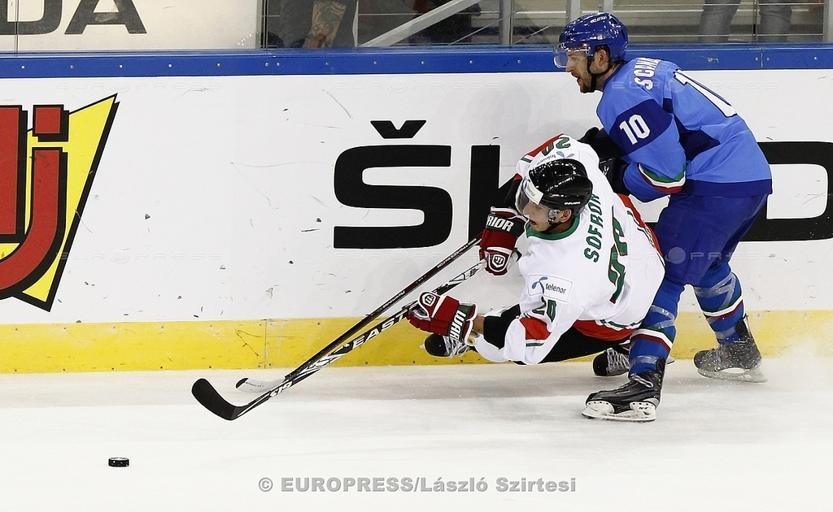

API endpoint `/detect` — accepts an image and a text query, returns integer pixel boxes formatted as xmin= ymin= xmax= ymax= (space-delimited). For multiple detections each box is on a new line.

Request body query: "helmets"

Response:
xmin=552 ymin=10 xmax=629 ymax=69
xmin=513 ymin=158 xmax=592 ymax=227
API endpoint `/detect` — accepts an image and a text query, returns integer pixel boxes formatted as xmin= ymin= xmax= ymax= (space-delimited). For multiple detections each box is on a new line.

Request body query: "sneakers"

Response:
xmin=585 ymin=338 xmax=664 ymax=408
xmin=424 ymin=333 xmax=472 ymax=357
xmin=693 ymin=330 xmax=762 ymax=371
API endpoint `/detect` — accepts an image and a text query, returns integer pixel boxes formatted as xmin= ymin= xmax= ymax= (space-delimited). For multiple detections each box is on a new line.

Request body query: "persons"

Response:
xmin=408 ymin=0 xmax=482 ymax=47
xmin=700 ymin=0 xmax=792 ymax=43
xmin=552 ymin=13 xmax=773 ymax=425
xmin=268 ymin=0 xmax=359 ymax=48
xmin=402 ymin=132 xmax=664 ymax=376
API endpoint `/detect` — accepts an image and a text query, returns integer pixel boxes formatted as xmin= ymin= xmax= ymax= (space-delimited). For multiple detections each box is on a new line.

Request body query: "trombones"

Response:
xmin=235 ymin=236 xmax=481 ymax=392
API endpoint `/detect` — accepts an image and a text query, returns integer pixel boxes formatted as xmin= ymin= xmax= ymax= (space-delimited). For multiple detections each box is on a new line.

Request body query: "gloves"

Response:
xmin=478 ymin=206 xmax=527 ymax=275
xmin=576 ymin=127 xmax=629 ymax=197
xmin=401 ymin=293 xmax=477 ymax=346
